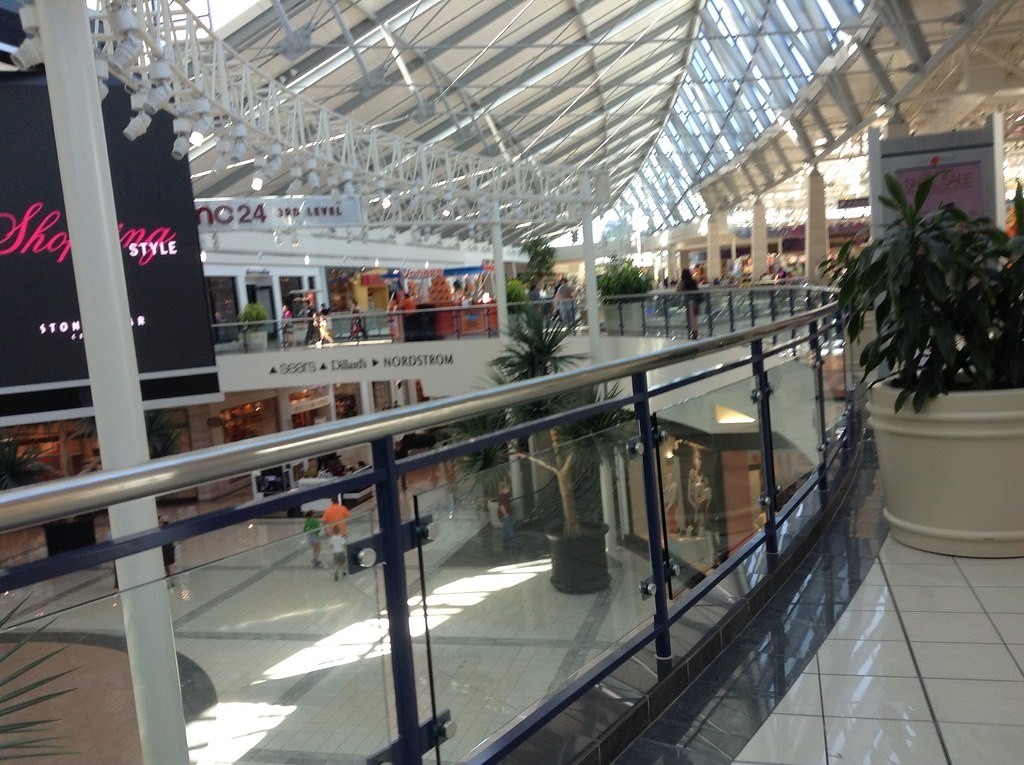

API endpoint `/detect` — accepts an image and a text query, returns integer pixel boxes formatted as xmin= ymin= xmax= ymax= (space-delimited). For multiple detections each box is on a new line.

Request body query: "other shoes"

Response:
xmin=690 ymin=330 xmax=698 ymax=339
xmin=334 ymin=570 xmax=347 ymax=581
xmin=311 ymin=557 xmax=321 ymax=566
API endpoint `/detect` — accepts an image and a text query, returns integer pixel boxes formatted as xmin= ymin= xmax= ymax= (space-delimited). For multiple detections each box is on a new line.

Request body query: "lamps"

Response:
xmin=10 ymin=0 xmax=576 ymax=247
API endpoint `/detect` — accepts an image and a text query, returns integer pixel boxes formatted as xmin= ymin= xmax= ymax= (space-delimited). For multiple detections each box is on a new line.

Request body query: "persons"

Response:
xmin=450 ymin=280 xmax=464 ymax=331
xmin=688 ymin=452 xmax=719 ymax=569
xmin=282 ymin=305 xmax=297 ymax=350
xmin=349 ymin=299 xmax=368 ymax=341
xmin=385 ymin=294 xmax=415 ymax=340
xmin=303 ymin=299 xmax=335 ymax=347
xmin=663 ymin=473 xmax=679 ymax=534
xmin=157 ymin=514 xmax=177 ymax=578
xmin=528 ymin=277 xmax=581 ymax=331
xmin=313 ymin=461 xmax=367 ymax=480
xmin=497 ymin=482 xmax=524 ymax=554
xmin=661 ymin=254 xmax=805 ymax=340
xmin=304 ymin=497 xmax=349 ymax=582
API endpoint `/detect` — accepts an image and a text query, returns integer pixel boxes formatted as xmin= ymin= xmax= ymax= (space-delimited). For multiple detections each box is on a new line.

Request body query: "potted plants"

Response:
xmin=506 ymin=280 xmax=529 ymax=334
xmin=0 ymin=407 xmax=189 ymax=557
xmin=442 ymin=301 xmax=634 ymax=592
xmin=817 ymin=161 xmax=1024 ymax=559
xmin=596 ymin=253 xmax=656 ymax=334
xmin=235 ymin=303 xmax=271 ymax=351
